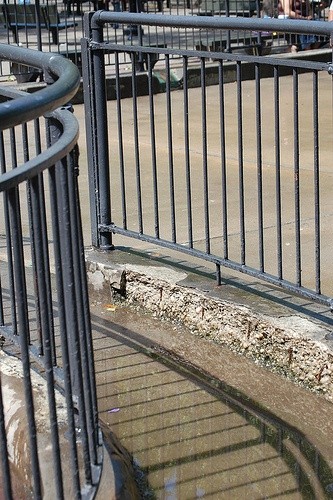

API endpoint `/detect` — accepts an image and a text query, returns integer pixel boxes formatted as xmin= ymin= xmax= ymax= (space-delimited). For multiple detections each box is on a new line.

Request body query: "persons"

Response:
xmin=293 ymin=1 xmax=316 ymax=51
xmin=280 ymin=0 xmax=324 ymax=53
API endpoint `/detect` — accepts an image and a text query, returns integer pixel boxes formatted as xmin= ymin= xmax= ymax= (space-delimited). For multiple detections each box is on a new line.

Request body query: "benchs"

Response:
xmin=47 ymin=41 xmax=168 ymax=75
xmin=5 ymin=23 xmax=77 ymax=44
xmin=197 ymin=31 xmax=284 ymax=59
xmin=188 ymin=11 xmax=258 ymax=16
